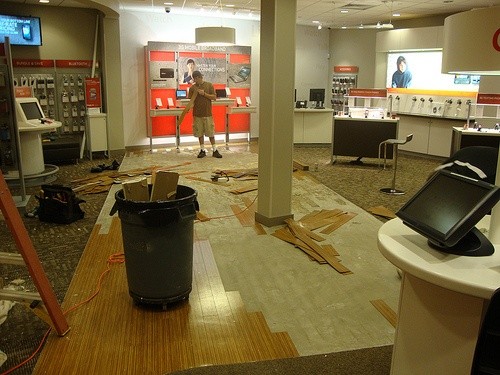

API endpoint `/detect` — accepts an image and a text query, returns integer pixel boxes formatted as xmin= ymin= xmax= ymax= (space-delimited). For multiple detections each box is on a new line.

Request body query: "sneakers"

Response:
xmin=197 ymin=149 xmax=206 ymax=158
xmin=213 ymin=150 xmax=222 ymax=158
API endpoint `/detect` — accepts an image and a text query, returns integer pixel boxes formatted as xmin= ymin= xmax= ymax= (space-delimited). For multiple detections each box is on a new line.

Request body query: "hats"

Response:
xmin=192 ymin=71 xmax=204 ymax=78
xmin=397 ymin=57 xmax=406 ymax=62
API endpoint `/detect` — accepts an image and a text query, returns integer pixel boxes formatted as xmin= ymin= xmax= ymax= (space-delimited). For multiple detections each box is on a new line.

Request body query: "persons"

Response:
xmin=189 ymin=71 xmax=223 ymax=158
xmin=183 ymin=59 xmax=196 ymax=84
xmin=392 ymin=56 xmax=412 ymax=88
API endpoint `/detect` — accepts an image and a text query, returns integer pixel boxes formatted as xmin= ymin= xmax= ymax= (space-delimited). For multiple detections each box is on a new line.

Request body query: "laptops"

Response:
xmin=229 ymin=66 xmax=250 ymax=83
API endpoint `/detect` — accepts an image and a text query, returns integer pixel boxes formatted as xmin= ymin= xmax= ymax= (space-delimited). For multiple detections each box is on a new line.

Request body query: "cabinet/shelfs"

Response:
xmin=151 ymin=98 xmax=257 ymax=117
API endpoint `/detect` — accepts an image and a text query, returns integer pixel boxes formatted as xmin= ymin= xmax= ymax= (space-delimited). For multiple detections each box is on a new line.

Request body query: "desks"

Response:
xmin=331 ymin=115 xmax=400 ymax=165
xmin=376 ymin=214 xmax=500 ymax=375
xmin=450 ymin=126 xmax=500 ymax=155
xmin=294 ymin=106 xmax=334 ymax=144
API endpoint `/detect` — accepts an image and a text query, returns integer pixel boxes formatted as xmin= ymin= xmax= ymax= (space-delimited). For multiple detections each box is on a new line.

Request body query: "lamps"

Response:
xmin=340 ymin=0 xmax=394 ymax=29
xmin=441 ymin=0 xmax=500 ymax=76
xmin=195 ymin=0 xmax=236 ymax=47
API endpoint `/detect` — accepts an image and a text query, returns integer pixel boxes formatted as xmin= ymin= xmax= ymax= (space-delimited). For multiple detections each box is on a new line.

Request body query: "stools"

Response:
xmin=379 ymin=133 xmax=413 ymax=195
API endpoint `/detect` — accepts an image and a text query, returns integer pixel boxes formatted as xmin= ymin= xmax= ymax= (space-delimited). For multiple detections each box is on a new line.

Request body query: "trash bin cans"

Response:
xmin=110 ymin=184 xmax=200 ymax=310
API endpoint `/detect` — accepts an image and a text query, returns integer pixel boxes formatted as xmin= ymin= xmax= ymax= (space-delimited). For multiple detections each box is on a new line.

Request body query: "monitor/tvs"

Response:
xmin=394 ymin=169 xmax=500 ymax=247
xmin=20 ymin=102 xmax=43 ymax=120
xmin=176 ymin=90 xmax=187 ymax=99
xmin=216 ymin=89 xmax=226 ymax=98
xmin=309 ymin=89 xmax=325 ymax=109
xmin=0 ymin=14 xmax=43 ymax=47
